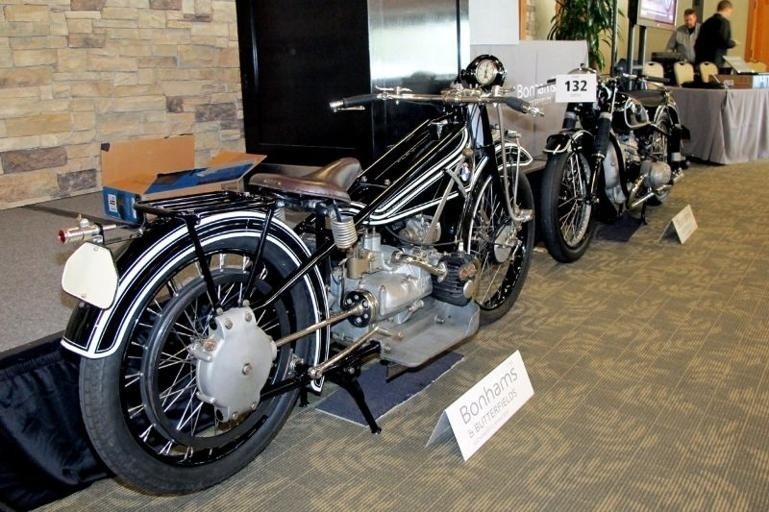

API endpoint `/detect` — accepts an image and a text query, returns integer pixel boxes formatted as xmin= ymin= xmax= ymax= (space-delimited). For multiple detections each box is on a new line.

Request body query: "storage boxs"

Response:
xmin=92 ymin=131 xmax=261 ymax=224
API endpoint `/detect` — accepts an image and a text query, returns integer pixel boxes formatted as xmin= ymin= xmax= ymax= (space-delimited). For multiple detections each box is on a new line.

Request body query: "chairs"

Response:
xmin=643 ymin=57 xmax=718 ymax=89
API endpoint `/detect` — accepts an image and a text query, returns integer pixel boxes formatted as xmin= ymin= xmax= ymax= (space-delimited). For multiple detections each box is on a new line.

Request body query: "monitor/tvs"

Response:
xmin=637 ymin=0 xmax=679 ymax=31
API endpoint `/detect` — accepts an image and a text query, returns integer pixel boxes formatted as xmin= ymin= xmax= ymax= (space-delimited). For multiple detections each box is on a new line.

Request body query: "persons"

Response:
xmin=664 ymin=0 xmax=742 ymax=75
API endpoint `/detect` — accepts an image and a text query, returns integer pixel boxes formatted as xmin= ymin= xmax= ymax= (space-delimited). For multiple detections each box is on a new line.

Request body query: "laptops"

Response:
xmin=722 ymin=55 xmax=769 ymax=75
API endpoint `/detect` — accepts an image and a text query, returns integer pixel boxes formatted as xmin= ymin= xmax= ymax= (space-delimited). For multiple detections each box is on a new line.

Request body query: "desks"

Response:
xmin=667 ymin=84 xmax=769 ymax=163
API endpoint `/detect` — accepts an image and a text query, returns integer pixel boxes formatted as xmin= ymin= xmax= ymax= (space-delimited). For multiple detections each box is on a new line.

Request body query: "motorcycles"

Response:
xmin=535 ymin=63 xmax=691 ymax=264
xmin=56 ymin=54 xmax=546 ymax=493
